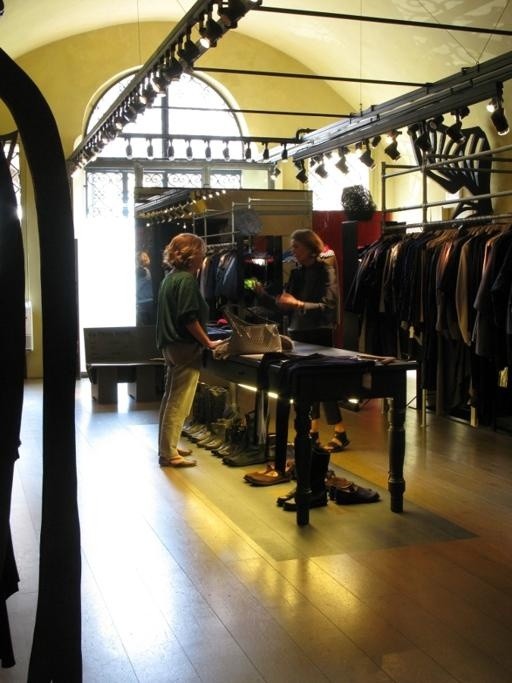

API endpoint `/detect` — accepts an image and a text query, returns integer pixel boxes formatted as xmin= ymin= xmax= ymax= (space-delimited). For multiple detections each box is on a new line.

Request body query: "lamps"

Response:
xmin=144 ymin=189 xmax=227 ymax=229
xmin=69 ymin=2 xmax=258 ymax=174
xmin=123 ymin=138 xmax=289 ymax=163
xmin=294 ymin=93 xmax=509 ymax=186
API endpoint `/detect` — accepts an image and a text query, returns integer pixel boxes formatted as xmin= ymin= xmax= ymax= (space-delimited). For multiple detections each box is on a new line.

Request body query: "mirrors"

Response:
xmin=134 ymin=187 xmax=313 ymax=326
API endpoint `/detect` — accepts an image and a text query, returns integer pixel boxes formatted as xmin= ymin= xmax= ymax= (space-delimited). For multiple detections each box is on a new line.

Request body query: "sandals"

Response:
xmin=319 ymin=431 xmax=350 ymax=452
xmin=158 ymin=455 xmax=197 ymax=467
xmin=308 ymin=432 xmax=321 ymax=445
xmin=174 ymin=446 xmax=193 ymax=456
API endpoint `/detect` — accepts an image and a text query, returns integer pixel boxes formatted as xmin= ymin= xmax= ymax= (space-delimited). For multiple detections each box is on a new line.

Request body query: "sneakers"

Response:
xmin=336 ymin=481 xmax=379 ymax=505
xmin=328 ymin=477 xmax=372 ymax=500
xmin=245 ymin=465 xmax=293 ymax=486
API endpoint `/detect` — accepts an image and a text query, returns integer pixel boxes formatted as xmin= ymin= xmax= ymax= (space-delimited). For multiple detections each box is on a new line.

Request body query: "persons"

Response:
xmin=154 ymin=231 xmax=228 ymax=469
xmin=133 ymin=250 xmax=158 ymax=325
xmin=249 ymin=227 xmax=349 ymax=453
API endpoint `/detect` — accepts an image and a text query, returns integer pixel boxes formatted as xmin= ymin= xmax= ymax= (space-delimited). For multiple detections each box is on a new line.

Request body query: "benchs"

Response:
xmin=83 ymin=326 xmax=166 ymax=404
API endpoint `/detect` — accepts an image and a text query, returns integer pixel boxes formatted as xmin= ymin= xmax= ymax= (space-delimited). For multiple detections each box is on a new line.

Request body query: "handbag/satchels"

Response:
xmin=222 ymin=310 xmax=282 ymax=353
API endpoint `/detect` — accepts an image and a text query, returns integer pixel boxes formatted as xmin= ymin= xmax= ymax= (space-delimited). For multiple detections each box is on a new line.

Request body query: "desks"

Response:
xmin=200 ymin=334 xmax=420 ymax=525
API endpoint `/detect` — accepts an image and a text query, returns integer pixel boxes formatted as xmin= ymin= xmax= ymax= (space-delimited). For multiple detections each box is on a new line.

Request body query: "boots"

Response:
xmin=230 ymin=410 xmax=271 ymax=467
xmin=284 ymin=446 xmax=329 ymax=511
xmin=277 ymin=438 xmax=320 ymax=506
xmin=267 ymin=432 xmax=294 ymax=460
xmin=223 ymin=414 xmax=250 ymax=465
xmin=181 ymin=380 xmax=227 ymax=442
xmin=197 ymin=411 xmax=247 ymax=456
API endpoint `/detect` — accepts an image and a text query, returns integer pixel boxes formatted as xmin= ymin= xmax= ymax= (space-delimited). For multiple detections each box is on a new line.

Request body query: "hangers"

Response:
xmin=404 ymin=214 xmax=507 ymax=248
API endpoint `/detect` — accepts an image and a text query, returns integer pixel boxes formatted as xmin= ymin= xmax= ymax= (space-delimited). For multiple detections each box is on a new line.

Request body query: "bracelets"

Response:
xmin=297 ymin=299 xmax=301 ymax=309
